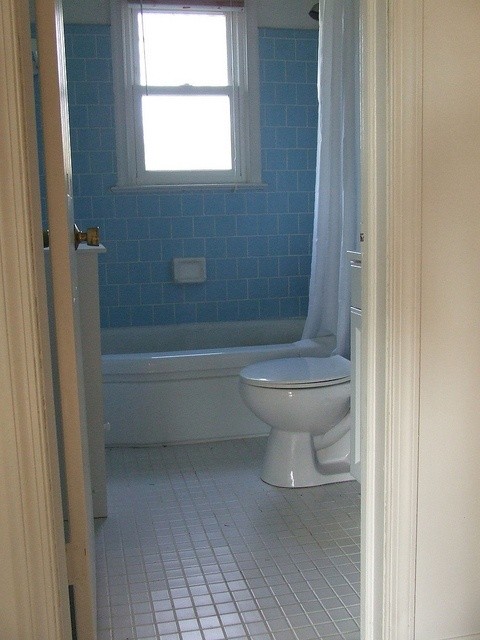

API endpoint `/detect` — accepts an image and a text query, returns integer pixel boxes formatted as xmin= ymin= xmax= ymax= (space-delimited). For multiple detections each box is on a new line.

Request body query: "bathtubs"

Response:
xmin=100 ymin=318 xmax=336 ymax=449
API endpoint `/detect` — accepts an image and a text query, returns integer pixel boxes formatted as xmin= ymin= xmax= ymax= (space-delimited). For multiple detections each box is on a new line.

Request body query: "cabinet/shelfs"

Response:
xmin=44 ymin=244 xmax=109 ymax=519
xmin=346 ymin=250 xmax=361 ymax=485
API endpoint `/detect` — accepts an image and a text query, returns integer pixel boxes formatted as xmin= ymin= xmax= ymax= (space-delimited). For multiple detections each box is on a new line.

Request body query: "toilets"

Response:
xmin=237 ymin=354 xmax=357 ymax=488
